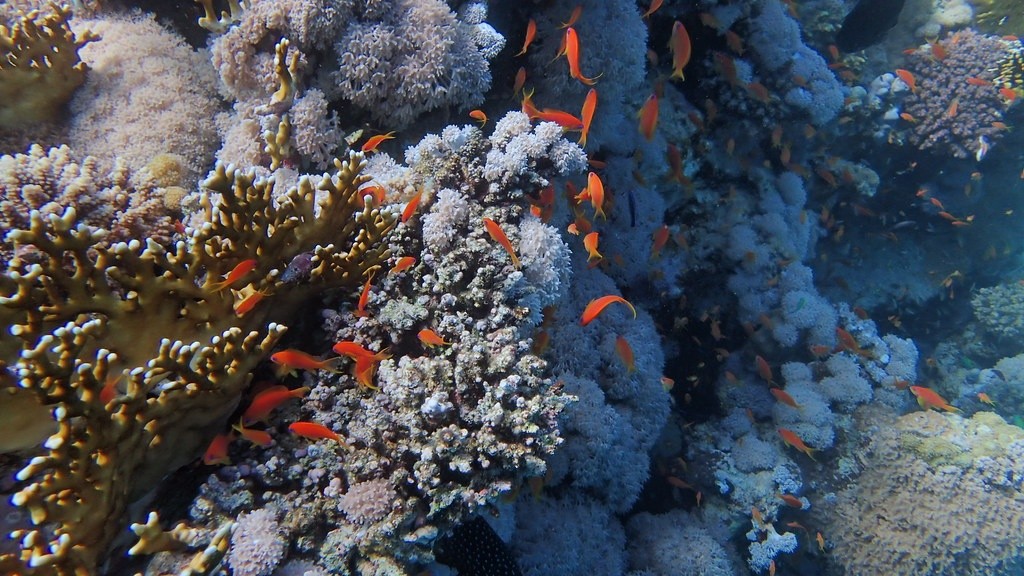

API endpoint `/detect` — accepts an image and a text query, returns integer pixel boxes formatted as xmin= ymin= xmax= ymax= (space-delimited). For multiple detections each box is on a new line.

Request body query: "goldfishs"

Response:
xmin=98 ymin=0 xmax=1024 ymax=576
